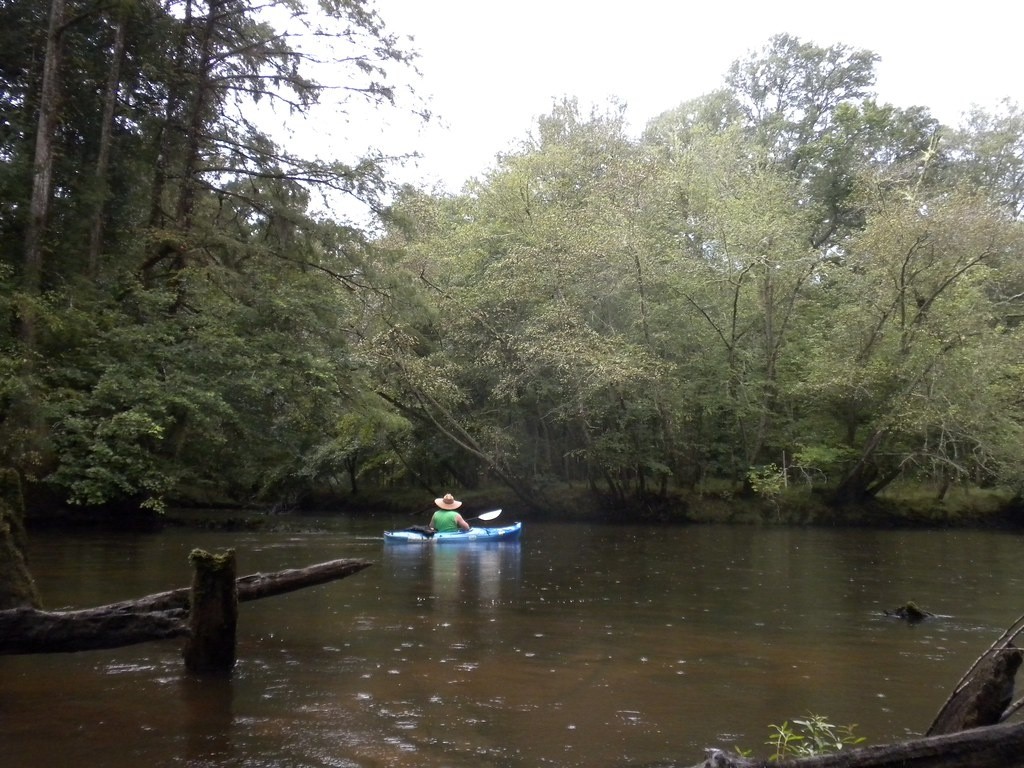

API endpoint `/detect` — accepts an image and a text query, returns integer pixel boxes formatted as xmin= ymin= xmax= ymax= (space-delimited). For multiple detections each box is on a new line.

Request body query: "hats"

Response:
xmin=435 ymin=494 xmax=462 ymax=510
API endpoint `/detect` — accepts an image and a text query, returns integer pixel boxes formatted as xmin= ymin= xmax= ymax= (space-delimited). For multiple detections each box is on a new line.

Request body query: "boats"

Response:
xmin=384 ymin=522 xmax=522 ymax=545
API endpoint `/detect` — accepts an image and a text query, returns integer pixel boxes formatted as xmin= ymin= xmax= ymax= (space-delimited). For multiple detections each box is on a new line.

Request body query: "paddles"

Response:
xmin=464 ymin=508 xmax=503 ymax=523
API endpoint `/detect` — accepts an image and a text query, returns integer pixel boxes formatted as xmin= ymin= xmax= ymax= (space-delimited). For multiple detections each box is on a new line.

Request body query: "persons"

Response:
xmin=429 ymin=493 xmax=470 ymax=533
